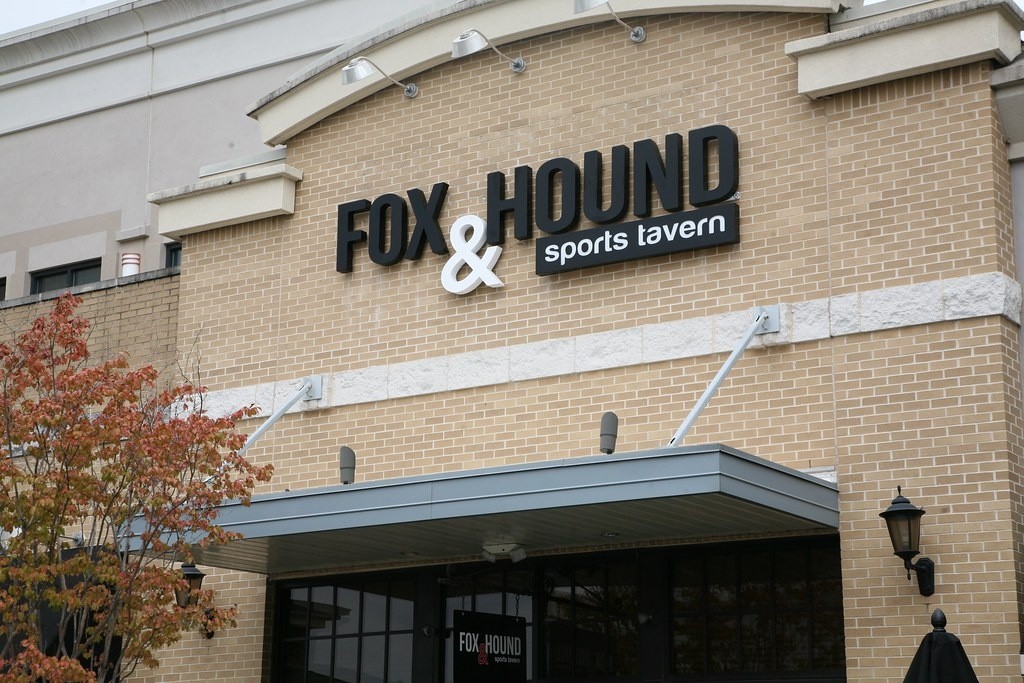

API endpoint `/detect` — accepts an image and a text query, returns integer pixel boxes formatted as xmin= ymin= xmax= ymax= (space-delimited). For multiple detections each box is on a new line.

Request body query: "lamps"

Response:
xmin=1 ymin=522 xmax=84 ymax=552
xmin=342 ymin=56 xmax=419 ymax=98
xmin=574 ymin=0 xmax=645 ymax=43
xmin=173 ymin=562 xmax=215 ymax=640
xmin=880 ymin=483 xmax=935 ymax=597
xmin=600 ymin=411 xmax=618 ymax=455
xmin=338 ymin=445 xmax=355 ymax=484
xmin=450 ymin=28 xmax=527 ymax=73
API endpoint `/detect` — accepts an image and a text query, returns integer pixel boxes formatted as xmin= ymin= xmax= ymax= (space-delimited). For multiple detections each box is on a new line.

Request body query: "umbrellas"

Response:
xmin=902 ymin=607 xmax=979 ymax=683
